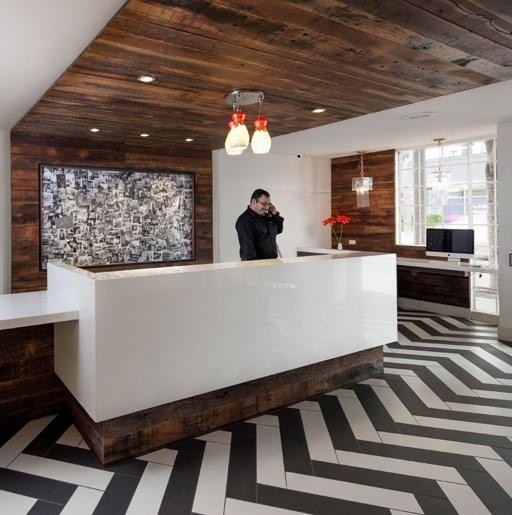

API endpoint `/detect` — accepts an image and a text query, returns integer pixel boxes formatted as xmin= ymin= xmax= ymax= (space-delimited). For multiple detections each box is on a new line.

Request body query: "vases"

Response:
xmin=338 ymin=242 xmax=342 ymax=250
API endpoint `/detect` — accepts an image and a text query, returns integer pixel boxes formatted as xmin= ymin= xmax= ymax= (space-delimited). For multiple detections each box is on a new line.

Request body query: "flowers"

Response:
xmin=322 ymin=215 xmax=351 ymax=243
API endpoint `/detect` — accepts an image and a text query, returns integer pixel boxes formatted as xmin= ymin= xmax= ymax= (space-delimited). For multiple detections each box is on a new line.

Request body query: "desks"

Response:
xmin=397 ymin=257 xmax=498 ymax=320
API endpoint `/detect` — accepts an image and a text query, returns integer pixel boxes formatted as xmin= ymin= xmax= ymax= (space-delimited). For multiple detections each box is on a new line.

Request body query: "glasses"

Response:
xmin=256 ymin=200 xmax=272 ymax=206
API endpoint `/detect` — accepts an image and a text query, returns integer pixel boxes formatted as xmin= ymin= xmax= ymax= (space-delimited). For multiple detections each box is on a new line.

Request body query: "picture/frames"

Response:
xmin=39 ymin=162 xmax=196 ymax=270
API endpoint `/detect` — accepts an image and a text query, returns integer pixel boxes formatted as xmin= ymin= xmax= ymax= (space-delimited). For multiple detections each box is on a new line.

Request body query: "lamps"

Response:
xmin=223 ymin=90 xmax=271 ymax=155
xmin=429 ymin=137 xmax=451 ymax=186
xmin=352 ymin=150 xmax=374 ymax=192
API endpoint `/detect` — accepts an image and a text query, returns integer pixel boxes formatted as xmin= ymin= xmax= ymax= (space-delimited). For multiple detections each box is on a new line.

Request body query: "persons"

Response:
xmin=235 ymin=189 xmax=285 ymax=261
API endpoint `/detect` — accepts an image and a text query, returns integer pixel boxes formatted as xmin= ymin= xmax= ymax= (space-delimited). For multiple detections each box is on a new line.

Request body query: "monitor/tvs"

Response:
xmin=425 ymin=227 xmax=475 ymax=264
